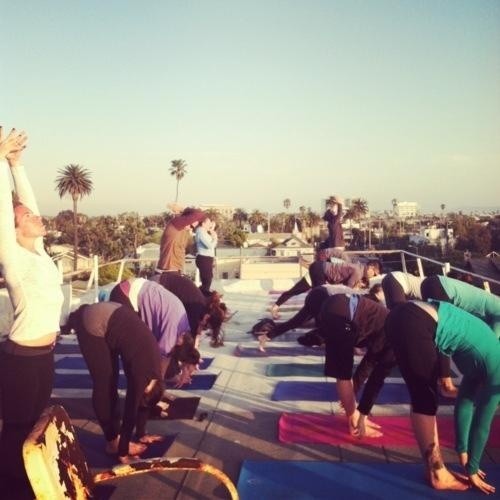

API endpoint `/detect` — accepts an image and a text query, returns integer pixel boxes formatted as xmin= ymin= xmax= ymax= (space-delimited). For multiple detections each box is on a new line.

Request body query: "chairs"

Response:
xmin=22 ymin=402 xmax=238 ymax=499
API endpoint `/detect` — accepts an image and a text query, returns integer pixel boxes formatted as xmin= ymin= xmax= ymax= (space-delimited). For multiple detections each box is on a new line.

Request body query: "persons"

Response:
xmin=64 ymin=300 xmax=166 ymax=466
xmin=326 ymin=200 xmax=345 ymax=249
xmin=384 ymin=300 xmax=499 ymax=494
xmin=0 ymin=121 xmax=65 ymax=499
xmin=321 ymin=292 xmax=398 ymax=436
xmin=259 ymin=248 xmax=423 ymax=355
xmin=194 ymin=212 xmax=217 ymax=297
xmin=421 ymin=274 xmax=500 ymax=397
xmin=156 ymin=206 xmax=209 ymax=275
xmin=149 ymin=271 xmax=227 ymax=389
xmin=108 ymin=276 xmax=201 ymax=419
